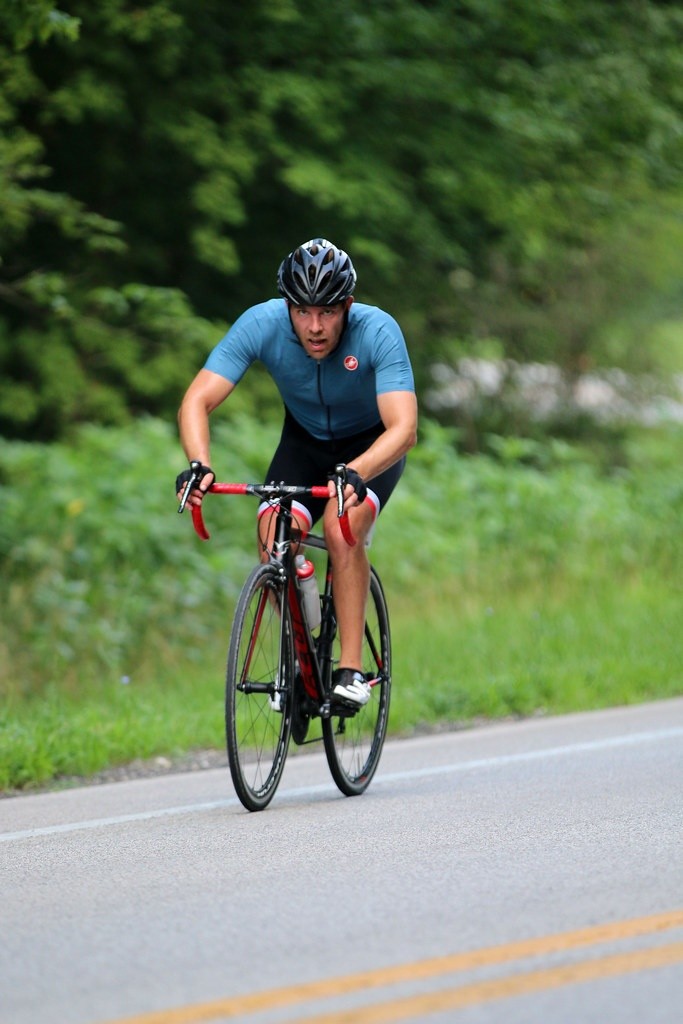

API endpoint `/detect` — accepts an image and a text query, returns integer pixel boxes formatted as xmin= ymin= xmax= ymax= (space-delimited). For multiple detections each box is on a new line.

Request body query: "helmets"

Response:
xmin=276 ymin=237 xmax=360 ymax=306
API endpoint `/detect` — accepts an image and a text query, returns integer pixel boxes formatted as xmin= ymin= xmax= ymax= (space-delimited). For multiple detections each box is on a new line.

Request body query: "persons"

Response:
xmin=178 ymin=238 xmax=418 ymax=714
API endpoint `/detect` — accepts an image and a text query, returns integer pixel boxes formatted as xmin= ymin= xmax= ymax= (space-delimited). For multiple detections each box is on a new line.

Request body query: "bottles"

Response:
xmin=297 ymin=555 xmax=321 ymax=629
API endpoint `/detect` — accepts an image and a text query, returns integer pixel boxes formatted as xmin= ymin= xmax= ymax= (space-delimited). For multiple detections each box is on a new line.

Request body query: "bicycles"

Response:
xmin=177 ymin=459 xmax=392 ymax=812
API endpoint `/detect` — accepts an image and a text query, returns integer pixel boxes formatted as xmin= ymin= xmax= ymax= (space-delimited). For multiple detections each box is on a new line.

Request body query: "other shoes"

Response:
xmin=331 ymin=667 xmax=373 ymax=709
xmin=269 ymin=660 xmax=299 ymax=711
